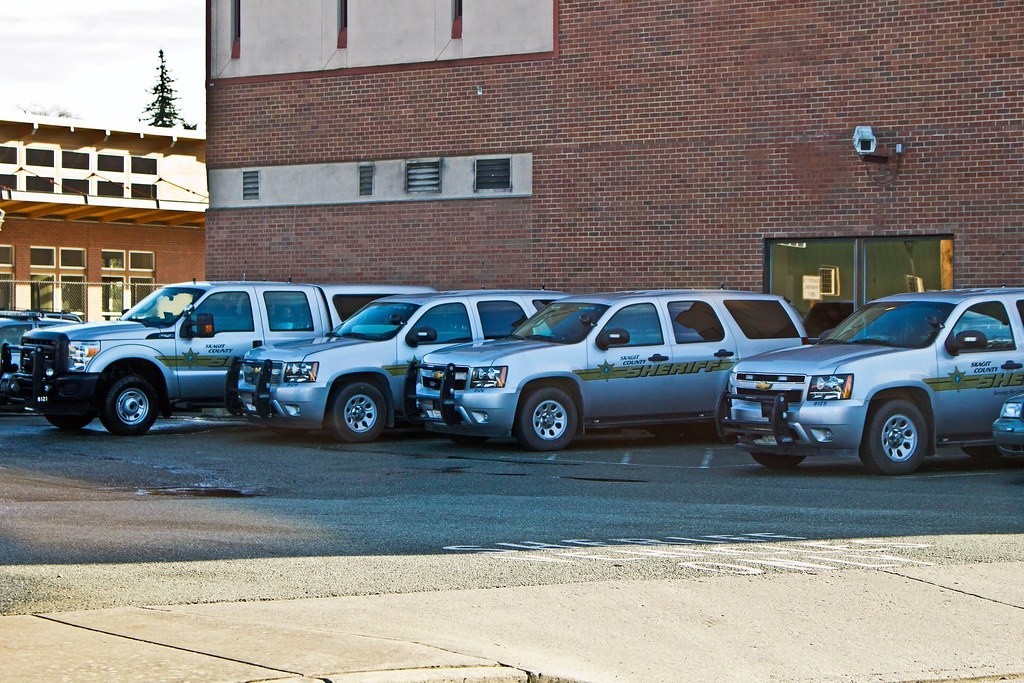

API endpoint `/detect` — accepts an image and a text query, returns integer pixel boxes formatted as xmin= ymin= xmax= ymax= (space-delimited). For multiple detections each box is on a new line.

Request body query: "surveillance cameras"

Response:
xmin=851 ymin=125 xmax=878 ymax=159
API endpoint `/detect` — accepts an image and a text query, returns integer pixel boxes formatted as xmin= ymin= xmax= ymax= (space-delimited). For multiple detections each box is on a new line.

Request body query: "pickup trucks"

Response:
xmin=6 ymin=277 xmax=443 ymax=438
xmin=224 ymin=287 xmax=583 ymax=447
xmin=399 ymin=287 xmax=811 ymax=454
xmin=717 ymin=283 xmax=1024 ymax=478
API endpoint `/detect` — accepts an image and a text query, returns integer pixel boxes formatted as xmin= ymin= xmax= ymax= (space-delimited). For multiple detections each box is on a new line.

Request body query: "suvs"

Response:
xmin=0 ymin=309 xmax=90 ymax=414
